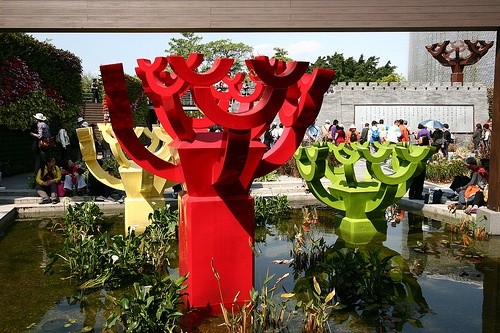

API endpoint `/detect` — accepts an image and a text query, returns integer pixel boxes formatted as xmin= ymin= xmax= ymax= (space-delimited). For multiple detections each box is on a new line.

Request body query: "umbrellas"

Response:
xmin=418 ymin=118 xmax=445 ymax=131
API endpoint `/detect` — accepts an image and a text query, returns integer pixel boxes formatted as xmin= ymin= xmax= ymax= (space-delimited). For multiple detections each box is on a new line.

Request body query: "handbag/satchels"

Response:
xmin=327 ymin=130 xmax=339 ymax=140
xmin=38 ymin=136 xmax=53 ymax=149
xmin=57 ymin=182 xmax=65 ymax=197
xmin=56 ymin=134 xmax=61 ymax=143
xmin=424 ymin=191 xmax=446 ymax=204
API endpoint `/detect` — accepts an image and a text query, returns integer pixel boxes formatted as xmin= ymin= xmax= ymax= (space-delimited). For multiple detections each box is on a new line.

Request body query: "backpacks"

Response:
xmin=349 ymin=130 xmax=357 ymax=142
xmin=369 ymin=128 xmax=381 ymax=144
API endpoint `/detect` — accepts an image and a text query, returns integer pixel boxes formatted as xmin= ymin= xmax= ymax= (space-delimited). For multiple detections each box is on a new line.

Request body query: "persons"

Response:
xmin=415 ymin=123 xmax=451 ymax=159
xmin=305 ymin=119 xmax=412 ymax=147
xmin=72 ymin=116 xmax=89 ymax=158
xmin=35 ymin=156 xmax=125 ymax=204
xmin=30 ymin=113 xmax=52 ymax=161
xmin=243 ymin=81 xmax=250 ymax=95
xmin=472 ymin=123 xmax=492 ymax=158
xmin=91 ymin=78 xmax=102 ymax=104
xmin=55 ymin=117 xmax=71 ymax=161
xmin=263 ymin=123 xmax=284 ymax=150
xmin=447 ymin=156 xmax=490 ymax=214
xmin=408 ymin=161 xmax=426 ymax=201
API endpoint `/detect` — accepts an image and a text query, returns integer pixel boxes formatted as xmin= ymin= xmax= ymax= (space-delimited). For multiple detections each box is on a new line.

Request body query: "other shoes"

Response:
xmin=463 ymin=207 xmax=477 ymax=213
xmin=53 ymin=198 xmax=58 ymax=203
xmin=39 ymin=198 xmax=52 ymax=204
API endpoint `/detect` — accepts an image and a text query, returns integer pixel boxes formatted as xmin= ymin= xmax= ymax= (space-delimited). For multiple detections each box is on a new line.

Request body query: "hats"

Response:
xmin=325 ymin=119 xmax=330 ymax=123
xmin=77 ymin=117 xmax=85 ymax=122
xmin=33 ymin=113 xmax=46 ymax=120
xmin=349 ymin=124 xmax=356 ymax=128
